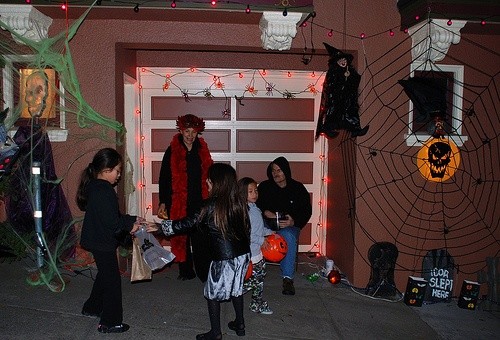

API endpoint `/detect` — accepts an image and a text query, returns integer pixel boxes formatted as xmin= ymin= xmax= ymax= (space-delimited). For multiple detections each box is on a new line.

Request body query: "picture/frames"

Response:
xmin=413 ymin=69 xmax=456 ymax=133
xmin=12 ymin=61 xmax=59 ymax=127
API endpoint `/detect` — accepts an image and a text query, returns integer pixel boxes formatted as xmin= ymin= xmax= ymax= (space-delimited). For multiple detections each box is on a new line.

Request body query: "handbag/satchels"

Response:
xmin=458 ymin=279 xmax=480 ymax=309
xmin=403 ymin=276 xmax=428 ymax=307
xmin=133 ymin=223 xmax=176 ymax=272
xmin=130 ymin=238 xmax=153 ymax=282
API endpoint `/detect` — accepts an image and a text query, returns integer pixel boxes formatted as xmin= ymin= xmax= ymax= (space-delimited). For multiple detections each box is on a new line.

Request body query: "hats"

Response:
xmin=175 ymin=114 xmax=206 ymax=135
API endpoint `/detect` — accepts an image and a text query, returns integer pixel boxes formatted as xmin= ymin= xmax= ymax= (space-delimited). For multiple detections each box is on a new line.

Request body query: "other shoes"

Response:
xmin=261 ymin=309 xmax=274 ymax=315
xmin=81 ymin=310 xmax=98 ymax=316
xmin=97 ymin=322 xmax=130 ymax=334
xmin=282 ymin=277 xmax=296 ymax=295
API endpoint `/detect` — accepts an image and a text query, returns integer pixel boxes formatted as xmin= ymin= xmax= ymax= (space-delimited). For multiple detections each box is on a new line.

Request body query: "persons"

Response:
xmin=158 ymin=114 xmax=214 ymax=281
xmin=0 ymin=126 xmax=76 ymax=262
xmin=76 ymin=147 xmax=145 ymax=334
xmin=255 ymin=157 xmax=312 ymax=295
xmin=239 ymin=177 xmax=277 ymax=314
xmin=146 ymin=162 xmax=255 ymax=340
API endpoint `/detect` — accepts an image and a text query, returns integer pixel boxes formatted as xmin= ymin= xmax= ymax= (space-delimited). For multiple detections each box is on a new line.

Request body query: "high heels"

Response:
xmin=228 ymin=320 xmax=246 ymax=336
xmin=196 ymin=329 xmax=222 ymax=340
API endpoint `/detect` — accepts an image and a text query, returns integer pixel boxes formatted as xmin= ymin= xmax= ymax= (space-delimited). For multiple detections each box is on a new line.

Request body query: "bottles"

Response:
xmin=325 ymin=259 xmax=334 ymax=277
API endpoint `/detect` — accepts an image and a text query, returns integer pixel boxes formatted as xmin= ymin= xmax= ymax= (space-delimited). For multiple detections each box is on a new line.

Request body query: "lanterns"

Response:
xmin=262 ymin=235 xmax=288 ymax=263
xmin=244 ymin=261 xmax=253 ymax=281
